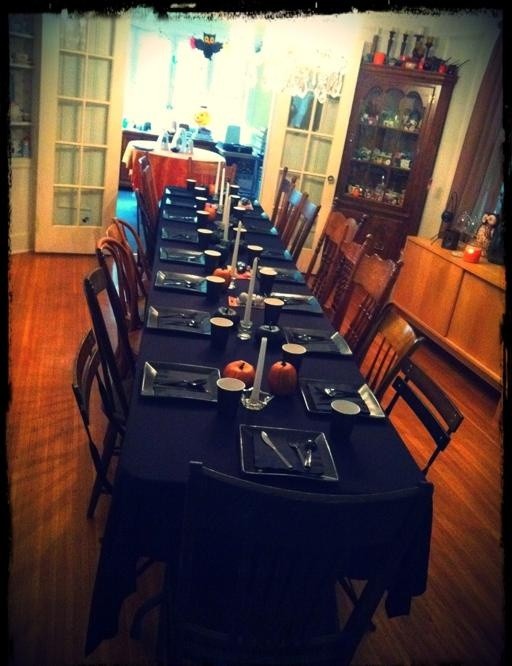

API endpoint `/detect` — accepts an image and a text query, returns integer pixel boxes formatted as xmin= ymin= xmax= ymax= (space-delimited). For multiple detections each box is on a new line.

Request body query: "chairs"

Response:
xmin=102 ymin=217 xmax=148 ymax=289
xmin=278 ymin=183 xmax=322 ymax=262
xmin=72 ymin=327 xmax=129 ymax=521
xmin=384 ymin=356 xmax=464 ymax=477
xmin=351 ymin=300 xmax=425 ymax=400
xmin=94 ymin=236 xmax=143 ymax=354
xmin=302 ymin=196 xmax=405 ymax=358
xmin=80 ymin=266 xmax=137 ymax=396
xmin=266 ymin=165 xmax=299 ymax=238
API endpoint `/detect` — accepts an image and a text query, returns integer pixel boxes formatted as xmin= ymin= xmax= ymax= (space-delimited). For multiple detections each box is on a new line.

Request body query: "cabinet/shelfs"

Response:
xmin=7 ymin=13 xmax=36 ymax=256
xmin=119 ymin=130 xmax=215 ymax=188
xmin=334 ymin=62 xmax=459 ymax=262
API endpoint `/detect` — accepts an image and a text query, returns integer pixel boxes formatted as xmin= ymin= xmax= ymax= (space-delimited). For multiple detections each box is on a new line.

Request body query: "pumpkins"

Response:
xmin=268 ymin=360 xmax=297 ymax=397
xmin=209 ymin=184 xmax=214 ymax=195
xmin=223 ymin=359 xmax=255 ymax=389
xmin=212 ymin=266 xmax=232 ymax=286
xmin=204 ymin=204 xmax=217 ymax=222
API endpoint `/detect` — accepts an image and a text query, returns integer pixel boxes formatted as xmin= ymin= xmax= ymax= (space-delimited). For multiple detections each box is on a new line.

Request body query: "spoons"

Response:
xmin=159 ymin=378 xmax=207 ymax=386
xmin=164 ymin=277 xmax=198 ymax=285
xmin=323 ymin=386 xmax=359 ymax=398
xmin=163 ymin=319 xmax=199 ymax=326
xmin=278 ymin=297 xmax=306 ymax=304
xmin=292 ymin=332 xmax=330 ymax=342
xmin=303 ymin=439 xmax=317 ymax=468
xmin=167 ymin=255 xmax=196 ymax=260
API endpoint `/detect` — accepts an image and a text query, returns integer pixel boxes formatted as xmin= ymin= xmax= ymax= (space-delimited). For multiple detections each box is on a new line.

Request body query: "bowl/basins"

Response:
xmin=405 ymin=62 xmax=417 ymax=69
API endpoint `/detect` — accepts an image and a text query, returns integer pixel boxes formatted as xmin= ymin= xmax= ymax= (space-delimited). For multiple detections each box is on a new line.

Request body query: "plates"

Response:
xmin=256 ymin=267 xmax=305 ymax=283
xmin=162 ymin=186 xmax=200 ymax=245
xmin=153 ymin=270 xmax=208 ymax=295
xmin=281 ymin=326 xmax=353 ymax=356
xmin=264 ymin=290 xmax=324 ymax=315
xmin=133 ymin=143 xmax=155 ymax=151
xmin=146 ymin=305 xmax=213 ymax=336
xmin=237 ymin=422 xmax=340 ymax=484
xmin=298 ymin=375 xmax=386 ymax=418
xmin=139 ymin=360 xmax=223 ymax=405
xmin=237 ymin=196 xmax=293 ymax=261
xmin=159 ymin=244 xmax=206 ymax=267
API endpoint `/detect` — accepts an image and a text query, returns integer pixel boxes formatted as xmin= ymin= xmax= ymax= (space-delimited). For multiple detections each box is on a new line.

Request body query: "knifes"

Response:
xmin=260 ymin=430 xmax=292 ymax=469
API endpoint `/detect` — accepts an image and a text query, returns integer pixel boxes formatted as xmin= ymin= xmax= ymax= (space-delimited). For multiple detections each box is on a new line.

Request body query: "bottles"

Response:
xmin=155 ymin=121 xmax=194 ymax=154
xmin=448 ymin=65 xmax=457 ymax=74
xmin=437 ymin=63 xmax=446 ymax=73
xmin=366 ymin=184 xmax=372 ymax=198
xmin=462 ymin=234 xmax=484 ymax=264
xmin=399 ymin=190 xmax=406 ymax=204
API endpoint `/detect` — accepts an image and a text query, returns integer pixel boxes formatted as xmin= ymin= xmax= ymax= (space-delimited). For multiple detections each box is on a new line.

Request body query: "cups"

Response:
xmin=442 ymin=227 xmax=459 ymax=250
xmin=263 ymin=298 xmax=285 ymax=325
xmin=186 ymin=178 xmax=247 ymax=249
xmin=216 ymin=377 xmax=245 ymax=425
xmin=205 ymin=275 xmax=224 ymax=301
xmin=281 ymin=342 xmax=307 ymax=371
xmin=373 ymin=50 xmax=386 ymax=65
xmin=204 ymin=250 xmax=222 ymax=274
xmin=15 ymin=52 xmax=33 ymax=61
xmin=259 ymin=266 xmax=276 ymax=294
xmin=209 ymin=318 xmax=234 ymax=349
xmin=248 ymin=245 xmax=263 ymax=268
xmin=399 ymin=56 xmax=409 ymax=67
xmin=331 ymin=399 xmax=361 ymax=444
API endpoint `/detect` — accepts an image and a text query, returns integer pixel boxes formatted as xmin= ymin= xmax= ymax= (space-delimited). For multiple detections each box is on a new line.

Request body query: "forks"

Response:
xmin=285 ymin=433 xmax=307 ymax=469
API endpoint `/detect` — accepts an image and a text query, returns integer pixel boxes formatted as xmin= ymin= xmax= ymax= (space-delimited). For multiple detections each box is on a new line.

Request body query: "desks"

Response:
xmin=393 ymin=235 xmax=506 ymax=428
xmin=111 ymin=183 xmax=435 ymax=665
